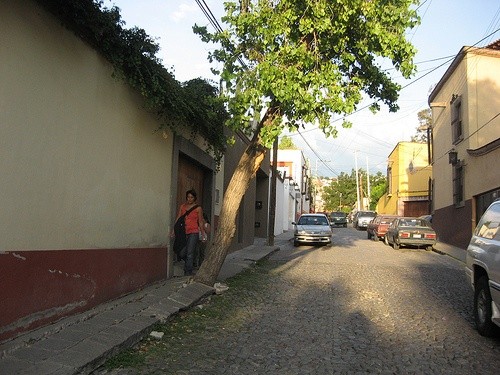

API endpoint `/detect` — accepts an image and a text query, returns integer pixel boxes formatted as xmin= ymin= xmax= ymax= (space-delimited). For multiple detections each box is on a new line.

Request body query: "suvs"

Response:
xmin=465 ymin=197 xmax=499 ymax=337
xmin=329 ymin=211 xmax=348 ymax=227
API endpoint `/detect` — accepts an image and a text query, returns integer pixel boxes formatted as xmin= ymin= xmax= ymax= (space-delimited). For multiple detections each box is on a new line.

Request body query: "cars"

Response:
xmin=352 ymin=211 xmax=378 ymax=230
xmin=385 ymin=217 xmax=436 ymax=251
xmin=366 ymin=215 xmax=404 ymax=242
xmin=291 ymin=214 xmax=335 ymax=246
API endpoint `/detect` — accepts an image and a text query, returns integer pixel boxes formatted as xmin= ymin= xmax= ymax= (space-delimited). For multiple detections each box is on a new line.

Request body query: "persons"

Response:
xmin=323 ymin=211 xmax=327 ymax=216
xmin=193 ymin=215 xmax=211 ymax=268
xmin=348 ymin=212 xmax=353 ymax=223
xmin=170 ymin=190 xmax=206 ymax=276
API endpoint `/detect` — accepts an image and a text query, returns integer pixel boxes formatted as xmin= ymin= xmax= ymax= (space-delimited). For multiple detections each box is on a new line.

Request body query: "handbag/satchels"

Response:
xmin=174 ymin=218 xmax=186 ymax=239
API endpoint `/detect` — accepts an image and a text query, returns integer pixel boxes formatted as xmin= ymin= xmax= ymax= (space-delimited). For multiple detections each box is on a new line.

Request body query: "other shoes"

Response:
xmin=185 ymin=271 xmax=192 ymax=275
